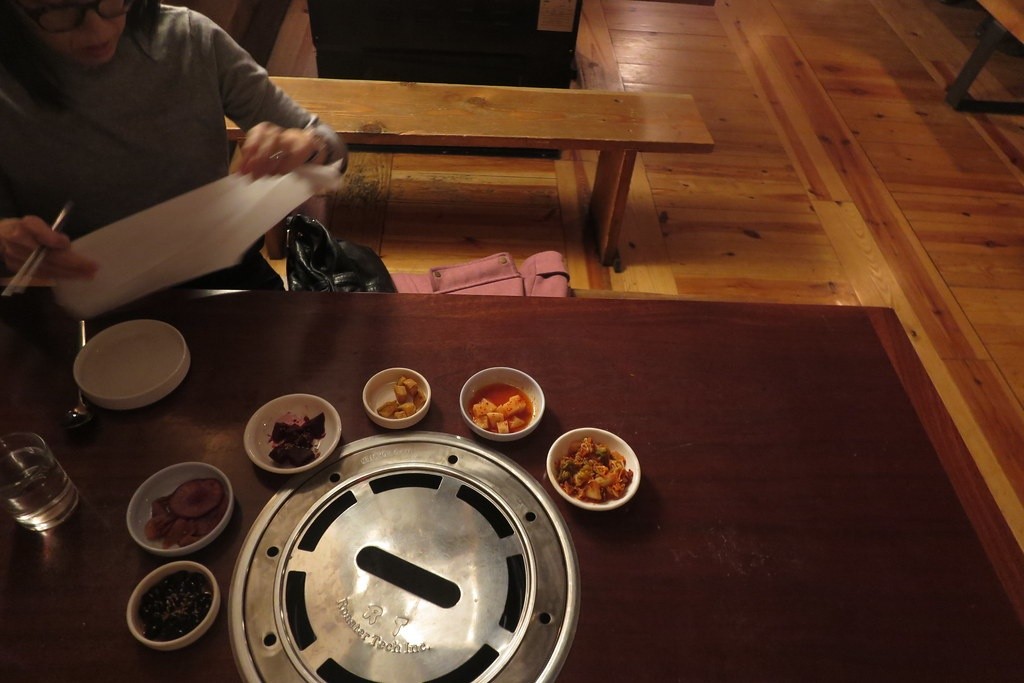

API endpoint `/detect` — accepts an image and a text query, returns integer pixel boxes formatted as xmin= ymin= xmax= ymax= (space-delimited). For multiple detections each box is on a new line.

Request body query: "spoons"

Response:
xmin=58 ymin=320 xmax=94 ymax=428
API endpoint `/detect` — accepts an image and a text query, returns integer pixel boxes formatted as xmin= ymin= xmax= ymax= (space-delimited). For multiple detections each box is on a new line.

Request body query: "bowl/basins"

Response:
xmin=362 ymin=368 xmax=431 ymax=430
xmin=73 ymin=319 xmax=190 ymax=410
xmin=458 ymin=366 xmax=545 ymax=441
xmin=125 ymin=462 xmax=235 ymax=557
xmin=126 ymin=561 xmax=221 ymax=651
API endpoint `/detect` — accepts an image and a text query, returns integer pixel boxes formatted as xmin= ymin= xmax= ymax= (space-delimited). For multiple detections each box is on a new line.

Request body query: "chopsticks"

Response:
xmin=0 ymin=202 xmax=73 ymax=297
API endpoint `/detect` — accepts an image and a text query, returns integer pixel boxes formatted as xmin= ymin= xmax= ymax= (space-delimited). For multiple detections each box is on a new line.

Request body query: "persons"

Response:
xmin=0 ymin=0 xmax=348 ymax=283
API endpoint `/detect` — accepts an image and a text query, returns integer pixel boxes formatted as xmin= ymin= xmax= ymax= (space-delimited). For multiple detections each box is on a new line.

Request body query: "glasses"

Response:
xmin=15 ymin=0 xmax=134 ymax=33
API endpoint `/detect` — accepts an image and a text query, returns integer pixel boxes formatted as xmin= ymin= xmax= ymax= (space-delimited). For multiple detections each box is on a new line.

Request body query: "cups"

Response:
xmin=0 ymin=430 xmax=80 ymax=531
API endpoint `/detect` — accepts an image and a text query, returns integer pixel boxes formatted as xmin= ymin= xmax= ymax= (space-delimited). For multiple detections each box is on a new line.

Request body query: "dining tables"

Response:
xmin=0 ymin=289 xmax=1024 ymax=683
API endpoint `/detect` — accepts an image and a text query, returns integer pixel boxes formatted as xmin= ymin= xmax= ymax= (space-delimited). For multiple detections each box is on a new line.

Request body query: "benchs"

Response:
xmin=222 ymin=75 xmax=715 ymax=265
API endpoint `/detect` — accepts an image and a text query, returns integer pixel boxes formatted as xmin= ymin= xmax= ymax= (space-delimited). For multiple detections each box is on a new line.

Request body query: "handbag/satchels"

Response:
xmin=286 ymin=213 xmax=398 ymax=293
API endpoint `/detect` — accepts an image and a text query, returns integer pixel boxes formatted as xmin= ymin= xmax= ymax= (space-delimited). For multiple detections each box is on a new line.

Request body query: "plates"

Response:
xmin=242 ymin=393 xmax=342 ymax=473
xmin=546 ymin=427 xmax=641 ymax=512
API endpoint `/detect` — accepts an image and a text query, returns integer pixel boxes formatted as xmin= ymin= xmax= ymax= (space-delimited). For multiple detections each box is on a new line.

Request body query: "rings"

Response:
xmin=269 ymin=151 xmax=285 ymax=164
